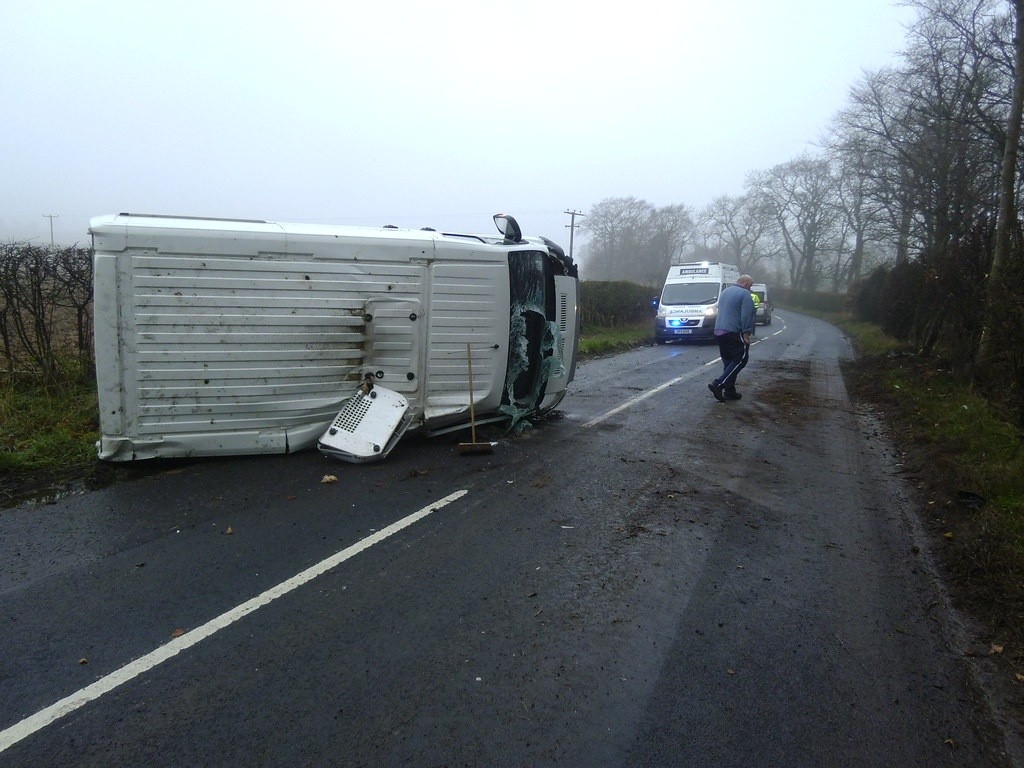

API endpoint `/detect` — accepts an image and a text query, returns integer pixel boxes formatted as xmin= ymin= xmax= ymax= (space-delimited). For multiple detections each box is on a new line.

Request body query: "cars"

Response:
xmin=755 ymin=300 xmax=773 ymax=324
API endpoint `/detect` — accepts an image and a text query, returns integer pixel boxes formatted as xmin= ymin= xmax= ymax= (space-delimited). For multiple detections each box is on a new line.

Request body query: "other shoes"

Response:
xmin=724 ymin=389 xmax=742 ymax=400
xmin=708 ymin=382 xmax=725 ymax=402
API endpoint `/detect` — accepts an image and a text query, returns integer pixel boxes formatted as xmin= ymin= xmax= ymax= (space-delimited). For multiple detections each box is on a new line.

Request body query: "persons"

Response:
xmin=707 ymin=275 xmax=756 ymax=401
xmin=750 ymin=289 xmax=760 ymax=337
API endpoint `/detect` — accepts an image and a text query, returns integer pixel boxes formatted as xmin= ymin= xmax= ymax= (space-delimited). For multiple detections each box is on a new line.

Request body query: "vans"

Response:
xmin=86 ymin=209 xmax=583 ymax=464
xmin=750 ymin=284 xmax=768 ymax=313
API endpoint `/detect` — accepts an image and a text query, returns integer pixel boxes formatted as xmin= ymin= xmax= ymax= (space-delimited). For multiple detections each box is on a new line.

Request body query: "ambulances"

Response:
xmin=649 ymin=261 xmax=737 ymax=344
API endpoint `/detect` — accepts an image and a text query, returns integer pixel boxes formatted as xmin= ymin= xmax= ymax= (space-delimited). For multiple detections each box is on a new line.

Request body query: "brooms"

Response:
xmin=457 ymin=343 xmax=493 ymax=452
